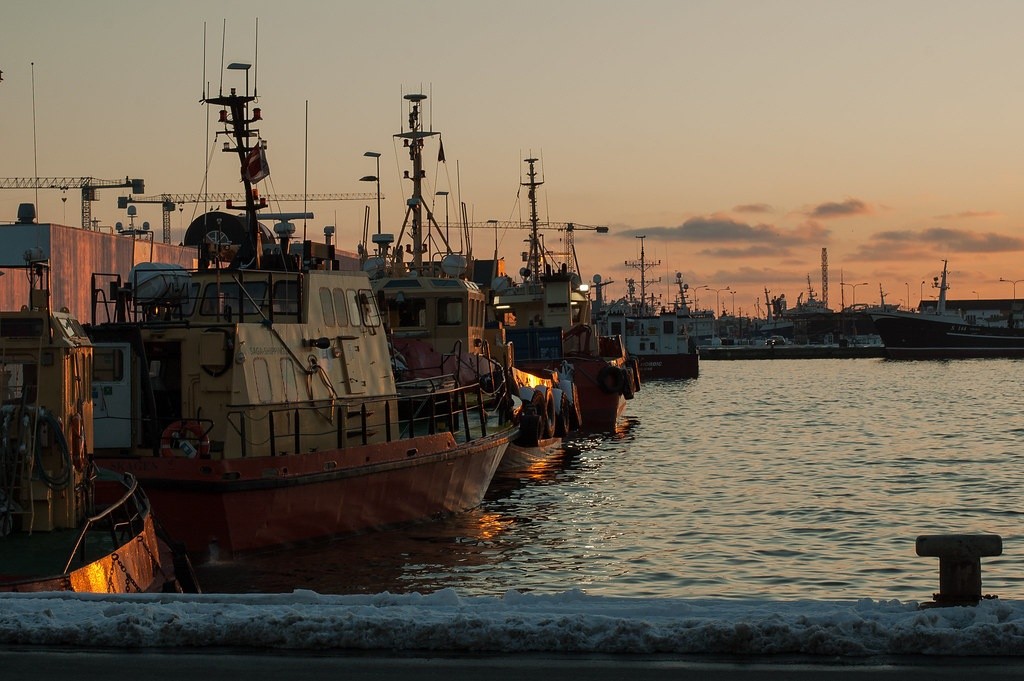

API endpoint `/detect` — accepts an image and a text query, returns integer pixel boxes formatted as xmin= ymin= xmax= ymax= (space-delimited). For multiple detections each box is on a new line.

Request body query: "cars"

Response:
xmin=766 ymin=336 xmax=785 ymax=345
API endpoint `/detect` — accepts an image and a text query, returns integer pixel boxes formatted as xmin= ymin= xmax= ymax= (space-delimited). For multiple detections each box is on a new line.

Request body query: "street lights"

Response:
xmin=360 ymin=151 xmax=382 ymax=252
xmin=840 ymin=282 xmax=868 ymax=311
xmin=706 ymin=287 xmax=731 ymax=338
xmin=999 ymin=279 xmax=1023 ymax=299
xmin=686 ymin=286 xmax=707 ymax=341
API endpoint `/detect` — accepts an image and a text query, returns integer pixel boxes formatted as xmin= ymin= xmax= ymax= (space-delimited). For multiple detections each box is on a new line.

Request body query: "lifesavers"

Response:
xmin=71 ymin=413 xmax=86 ymax=472
xmin=597 ymin=366 xmax=625 ymax=395
xmin=518 ymin=379 xmax=582 ymax=447
xmin=161 ymin=421 xmax=210 ymax=461
xmin=622 ymin=356 xmax=642 ymax=401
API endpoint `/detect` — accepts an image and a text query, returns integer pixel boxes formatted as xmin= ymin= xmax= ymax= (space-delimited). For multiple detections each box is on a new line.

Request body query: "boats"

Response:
xmin=94 ymin=57 xmax=643 ymax=551
xmin=1 ymin=265 xmax=171 ymax=595
xmin=868 ymin=258 xmax=1024 ymax=348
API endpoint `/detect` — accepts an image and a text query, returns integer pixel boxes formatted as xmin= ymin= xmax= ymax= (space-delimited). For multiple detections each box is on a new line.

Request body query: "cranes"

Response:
xmin=117 ymin=193 xmax=387 ymax=242
xmin=405 ymin=216 xmax=608 ymax=274
xmin=3 ymin=176 xmax=144 ymax=230
